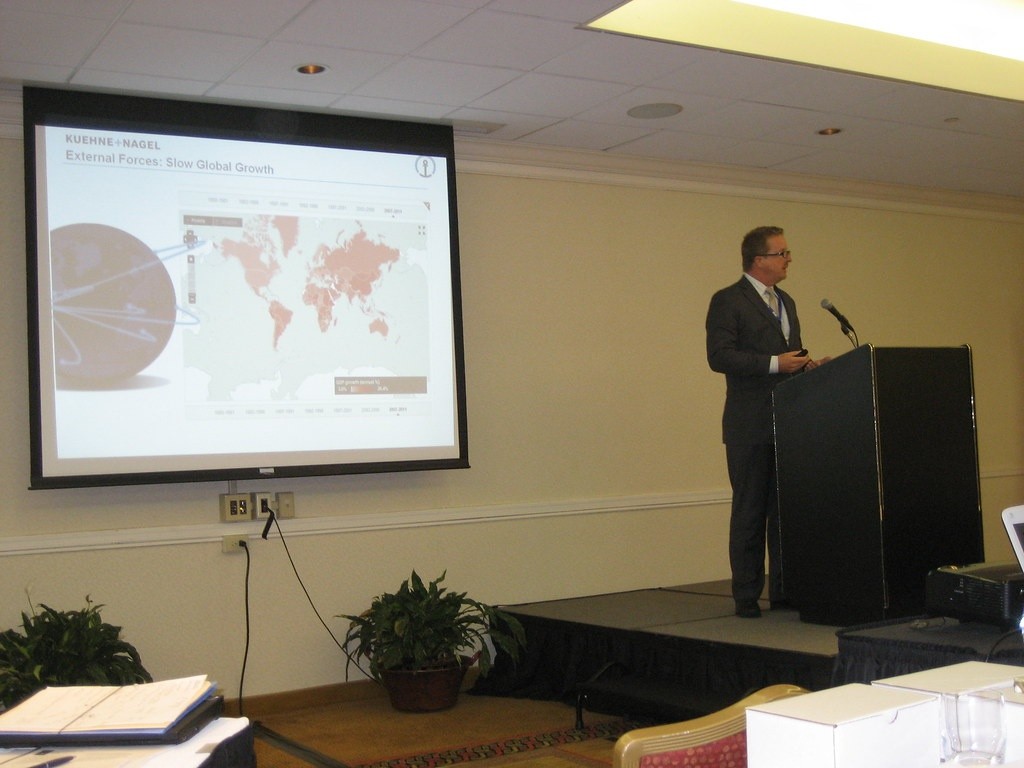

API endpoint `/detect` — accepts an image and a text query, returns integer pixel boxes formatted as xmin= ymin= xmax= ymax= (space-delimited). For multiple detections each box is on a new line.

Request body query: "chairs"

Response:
xmin=613 ymin=684 xmax=811 ymax=768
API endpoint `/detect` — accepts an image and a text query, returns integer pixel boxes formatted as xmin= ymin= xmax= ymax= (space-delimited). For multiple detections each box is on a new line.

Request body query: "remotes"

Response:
xmin=796 ymin=349 xmax=808 ymax=357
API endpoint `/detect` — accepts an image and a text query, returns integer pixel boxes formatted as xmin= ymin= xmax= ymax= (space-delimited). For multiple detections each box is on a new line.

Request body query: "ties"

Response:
xmin=766 ymin=287 xmax=780 ymax=318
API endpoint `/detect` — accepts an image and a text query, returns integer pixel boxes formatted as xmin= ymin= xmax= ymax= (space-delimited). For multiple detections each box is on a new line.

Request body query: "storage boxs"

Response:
xmin=746 ymin=661 xmax=1024 ymax=768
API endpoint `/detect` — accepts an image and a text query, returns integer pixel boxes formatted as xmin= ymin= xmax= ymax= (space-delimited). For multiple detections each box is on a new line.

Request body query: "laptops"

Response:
xmin=1001 ymin=504 xmax=1024 ymax=574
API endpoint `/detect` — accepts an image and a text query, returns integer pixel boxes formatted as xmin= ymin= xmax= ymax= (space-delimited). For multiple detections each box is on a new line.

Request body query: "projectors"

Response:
xmin=926 ymin=559 xmax=1024 ymax=632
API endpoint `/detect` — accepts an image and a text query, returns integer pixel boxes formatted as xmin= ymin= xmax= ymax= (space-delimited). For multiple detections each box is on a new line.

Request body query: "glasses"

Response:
xmin=757 ymin=250 xmax=790 ymax=258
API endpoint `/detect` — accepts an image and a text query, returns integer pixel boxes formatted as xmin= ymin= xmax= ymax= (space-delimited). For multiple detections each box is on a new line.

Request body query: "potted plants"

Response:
xmin=332 ymin=569 xmax=527 ymax=712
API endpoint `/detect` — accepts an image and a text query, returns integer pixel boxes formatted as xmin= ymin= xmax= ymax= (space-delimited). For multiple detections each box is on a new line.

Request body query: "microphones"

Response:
xmin=821 ymin=298 xmax=855 ymax=331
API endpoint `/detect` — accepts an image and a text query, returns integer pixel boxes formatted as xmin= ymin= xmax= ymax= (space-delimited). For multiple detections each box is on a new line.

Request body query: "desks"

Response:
xmin=0 ymin=717 xmax=258 ymax=768
xmin=834 ymin=613 xmax=1024 ymax=690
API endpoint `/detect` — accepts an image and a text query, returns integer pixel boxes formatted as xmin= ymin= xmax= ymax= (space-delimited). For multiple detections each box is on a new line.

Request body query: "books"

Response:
xmin=0 ymin=675 xmax=217 ymax=740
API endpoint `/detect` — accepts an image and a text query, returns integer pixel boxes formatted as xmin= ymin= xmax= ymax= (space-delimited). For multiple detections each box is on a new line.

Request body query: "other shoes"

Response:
xmin=736 ymin=602 xmax=762 ymax=618
xmin=770 ymin=600 xmax=788 ymax=611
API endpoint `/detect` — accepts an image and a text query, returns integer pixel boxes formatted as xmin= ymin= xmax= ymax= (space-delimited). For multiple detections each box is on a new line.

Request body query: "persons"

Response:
xmin=705 ymin=227 xmax=831 ymax=617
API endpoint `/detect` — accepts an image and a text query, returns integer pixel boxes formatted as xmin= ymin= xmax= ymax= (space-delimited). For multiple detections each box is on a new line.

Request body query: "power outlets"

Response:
xmin=250 ymin=492 xmax=271 ymax=520
xmin=223 ymin=534 xmax=249 ymax=552
xmin=220 ymin=494 xmax=251 ymax=522
